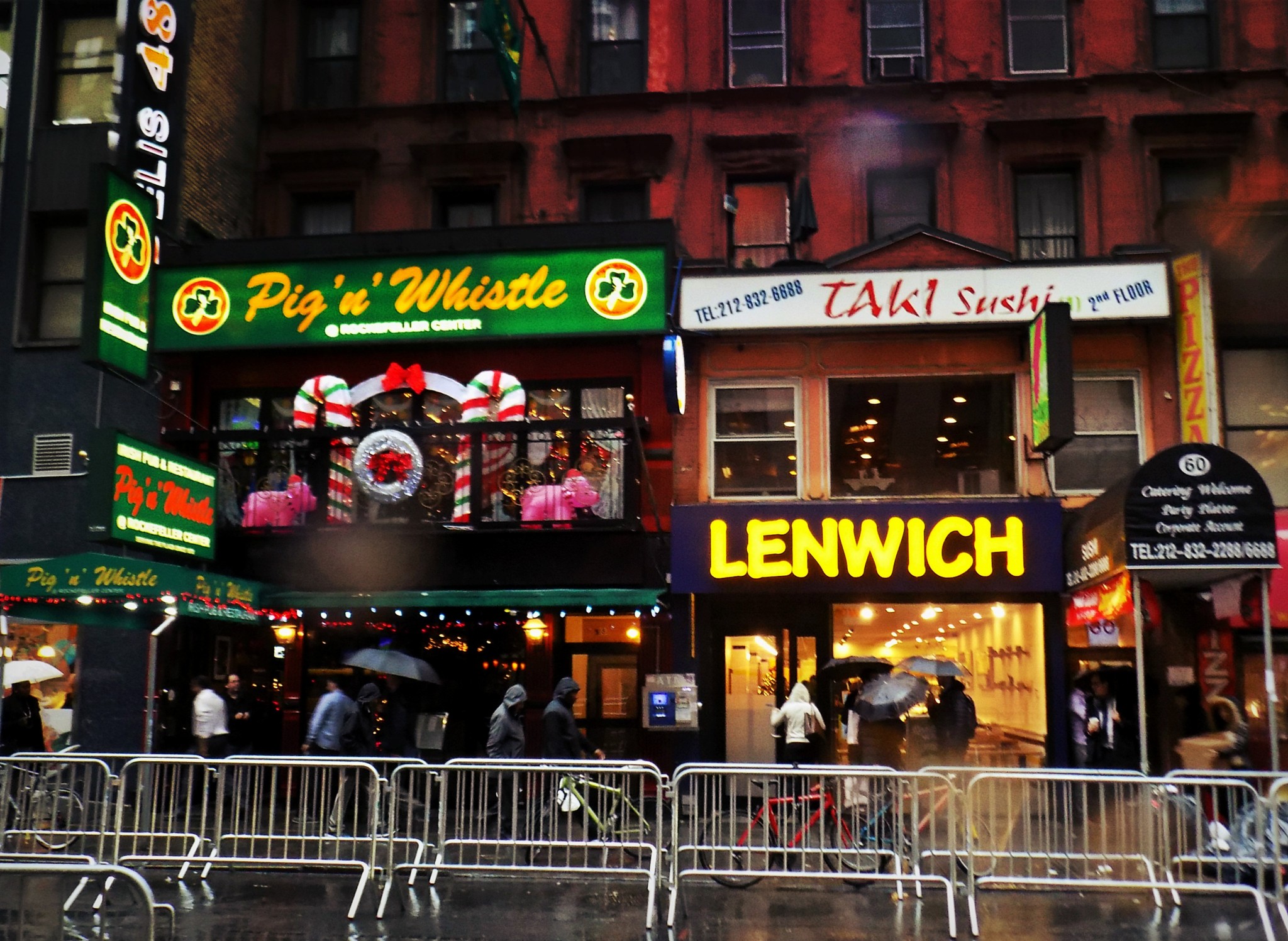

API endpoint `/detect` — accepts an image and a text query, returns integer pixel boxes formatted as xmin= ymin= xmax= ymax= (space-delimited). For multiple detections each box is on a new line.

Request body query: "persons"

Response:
xmin=164 ymin=672 xmax=230 ymax=821
xmin=293 ymin=674 xmax=359 ymax=823
xmin=924 ymin=673 xmax=978 ymax=767
xmin=209 ymin=673 xmax=252 ymax=803
xmin=478 ymin=683 xmax=527 ymax=838
xmin=856 ymin=716 xmax=907 ymax=826
xmin=1069 ymin=669 xmax=1254 ymax=817
xmin=520 ymin=676 xmax=606 ymax=843
xmin=0 ymin=680 xmax=46 ymax=837
xmin=772 ymin=669 xmax=828 ymax=826
xmin=328 ymin=681 xmax=397 ymax=838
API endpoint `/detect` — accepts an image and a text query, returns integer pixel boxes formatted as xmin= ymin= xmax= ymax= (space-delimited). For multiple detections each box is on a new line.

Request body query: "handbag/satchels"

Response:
xmin=804 ymin=703 xmax=826 ymax=744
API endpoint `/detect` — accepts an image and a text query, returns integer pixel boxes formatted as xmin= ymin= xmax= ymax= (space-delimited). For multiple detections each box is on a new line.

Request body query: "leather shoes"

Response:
xmin=164 ymin=809 xmax=186 ymax=821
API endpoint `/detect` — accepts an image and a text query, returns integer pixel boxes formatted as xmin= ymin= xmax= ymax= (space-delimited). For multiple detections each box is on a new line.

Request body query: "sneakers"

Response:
xmin=366 ymin=828 xmax=398 ymax=838
xmin=320 ymin=829 xmax=348 ymax=840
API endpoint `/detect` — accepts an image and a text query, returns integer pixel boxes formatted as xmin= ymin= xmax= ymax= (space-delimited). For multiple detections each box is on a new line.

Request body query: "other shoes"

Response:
xmin=477 ymin=812 xmax=489 ymax=829
xmin=501 ymin=830 xmax=514 ymax=841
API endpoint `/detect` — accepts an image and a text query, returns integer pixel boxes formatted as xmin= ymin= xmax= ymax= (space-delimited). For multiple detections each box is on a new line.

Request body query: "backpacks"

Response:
xmin=957 ymin=692 xmax=978 ymax=741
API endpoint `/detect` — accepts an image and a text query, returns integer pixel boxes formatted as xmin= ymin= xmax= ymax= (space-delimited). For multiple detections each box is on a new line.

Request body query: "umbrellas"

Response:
xmin=893 ymin=655 xmax=974 ymax=698
xmin=852 ymin=671 xmax=926 ymax=721
xmin=3 ymin=660 xmax=65 ymax=690
xmin=342 ymin=647 xmax=441 ymax=684
xmin=822 ymin=655 xmax=894 ymax=680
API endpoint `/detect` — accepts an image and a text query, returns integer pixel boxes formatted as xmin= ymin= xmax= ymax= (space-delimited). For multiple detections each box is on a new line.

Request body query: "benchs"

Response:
xmin=963 ymin=722 xmax=1045 ymax=771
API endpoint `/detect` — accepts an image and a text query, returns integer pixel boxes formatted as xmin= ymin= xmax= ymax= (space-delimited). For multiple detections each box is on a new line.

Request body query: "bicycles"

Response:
xmin=524 ymin=773 xmax=677 ymax=862
xmin=0 ymin=758 xmax=83 ymax=850
xmin=694 ymin=773 xmax=1000 ymax=889
